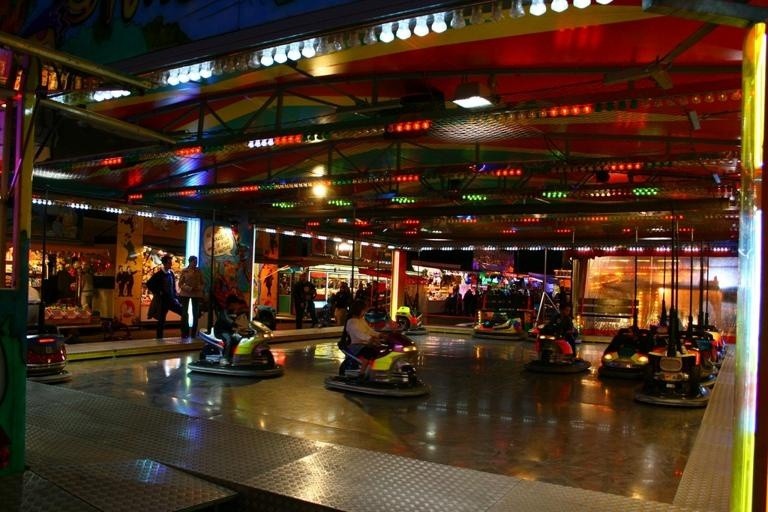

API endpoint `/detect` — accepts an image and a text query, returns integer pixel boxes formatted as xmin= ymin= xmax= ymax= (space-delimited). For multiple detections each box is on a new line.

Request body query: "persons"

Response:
xmin=344 ymin=298 xmax=390 ymax=382
xmin=145 ymin=254 xmax=183 ymax=339
xmin=215 ymin=294 xmax=242 ymax=368
xmin=538 ymin=300 xmax=578 ymax=358
xmin=115 ymin=265 xmax=128 ymax=297
xmin=177 ymin=255 xmax=205 ymax=338
xmin=293 ymin=271 xmax=319 ymax=328
xmin=439 ymin=283 xmax=568 ymax=317
xmin=320 ymin=278 xmax=390 ymax=327
xmin=262 ymin=268 xmax=275 ymax=298
xmin=126 ymin=266 xmax=142 ymax=297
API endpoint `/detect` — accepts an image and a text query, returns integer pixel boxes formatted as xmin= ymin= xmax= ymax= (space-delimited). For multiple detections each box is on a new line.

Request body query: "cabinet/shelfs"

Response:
xmin=424 ymin=284 xmax=450 ymax=299
xmin=279 ymin=270 xmax=388 ymax=314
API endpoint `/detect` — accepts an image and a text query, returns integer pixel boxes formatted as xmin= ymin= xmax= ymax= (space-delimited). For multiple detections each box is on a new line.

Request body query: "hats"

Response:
xmin=226 ymin=295 xmax=245 ymax=304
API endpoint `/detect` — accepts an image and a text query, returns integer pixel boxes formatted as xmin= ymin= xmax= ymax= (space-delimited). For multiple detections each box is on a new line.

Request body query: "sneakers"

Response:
xmin=219 ymin=358 xmax=229 ymax=366
